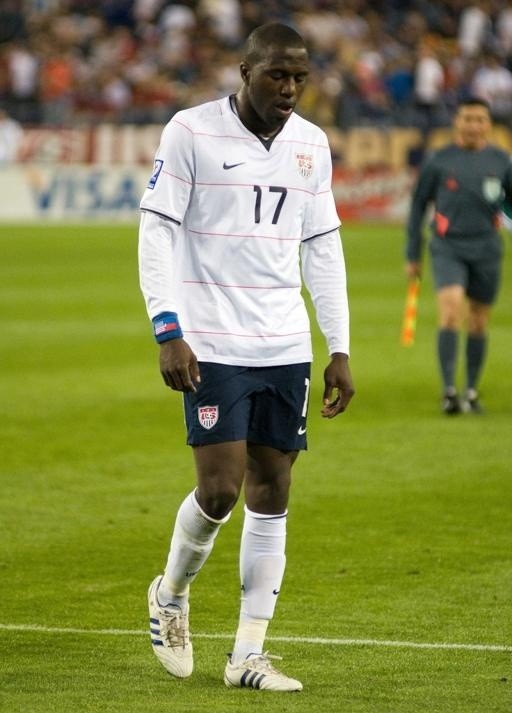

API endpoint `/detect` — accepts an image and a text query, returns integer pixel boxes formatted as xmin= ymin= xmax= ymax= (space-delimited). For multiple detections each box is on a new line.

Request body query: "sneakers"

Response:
xmin=467 ymin=392 xmax=481 ymax=411
xmin=223 ymin=653 xmax=304 ymax=694
xmin=146 ymin=572 xmax=195 ymax=677
xmin=443 ymin=392 xmax=462 ymax=413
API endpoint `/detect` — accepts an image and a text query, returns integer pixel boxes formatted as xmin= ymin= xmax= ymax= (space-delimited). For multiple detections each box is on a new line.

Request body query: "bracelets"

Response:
xmin=153 ymin=311 xmax=183 ymax=344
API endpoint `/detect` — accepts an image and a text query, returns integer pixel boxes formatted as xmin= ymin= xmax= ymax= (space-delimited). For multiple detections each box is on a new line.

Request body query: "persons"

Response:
xmin=403 ymin=94 xmax=511 ymax=415
xmin=1 ymin=0 xmax=511 ymax=189
xmin=134 ymin=21 xmax=358 ymax=692
